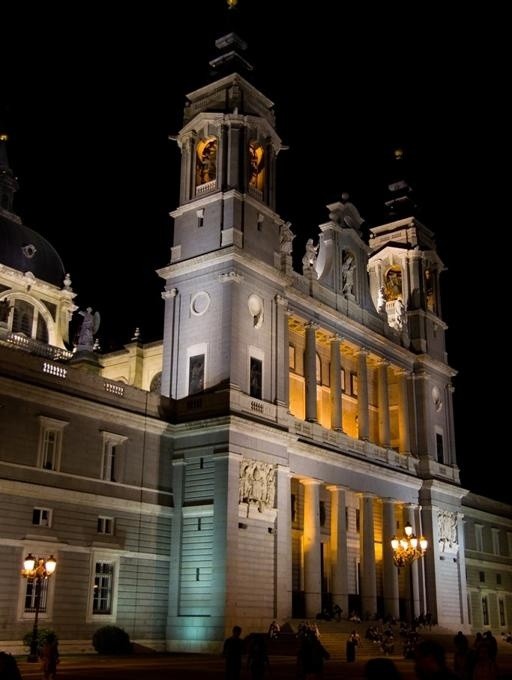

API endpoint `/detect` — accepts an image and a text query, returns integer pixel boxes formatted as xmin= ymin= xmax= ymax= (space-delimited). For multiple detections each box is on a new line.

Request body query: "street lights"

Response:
xmin=391 ymin=521 xmax=428 ymax=638
xmin=24 ymin=552 xmax=57 ymax=663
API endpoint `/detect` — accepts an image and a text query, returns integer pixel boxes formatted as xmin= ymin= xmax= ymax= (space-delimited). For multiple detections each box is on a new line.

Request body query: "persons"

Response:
xmin=39 ymin=633 xmax=59 ymax=680
xmin=76 ymin=307 xmax=103 ymax=346
xmin=265 ymin=468 xmax=275 ymax=504
xmin=342 ymin=253 xmax=357 ymax=302
xmin=302 ymin=239 xmax=320 ymax=282
xmin=0 ymin=651 xmax=23 ymax=679
xmin=221 ymin=604 xmax=512 ymax=680
